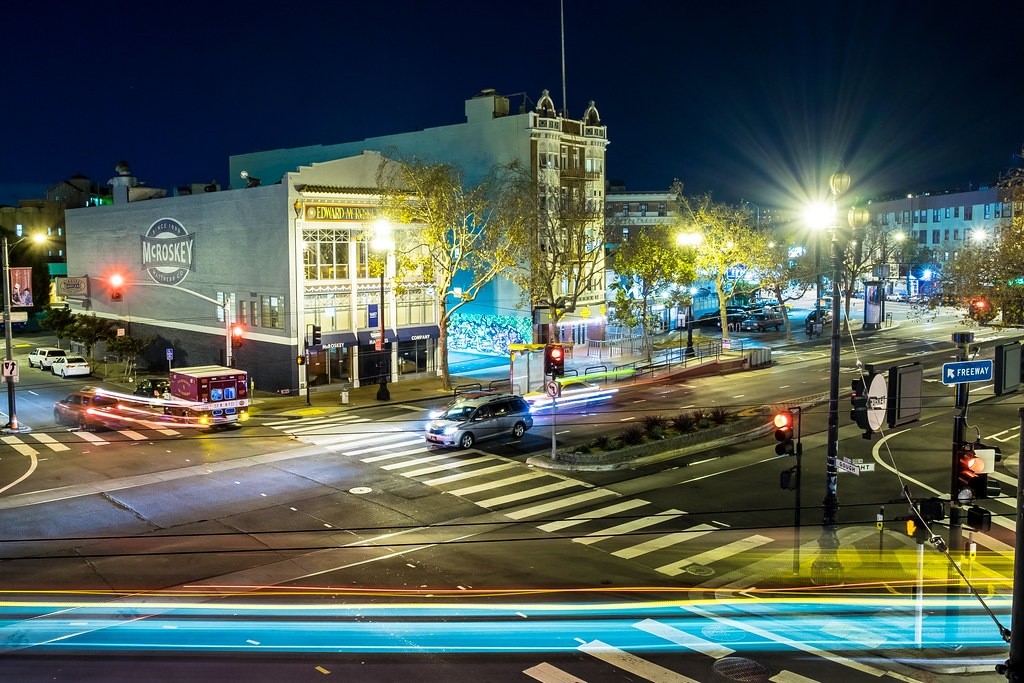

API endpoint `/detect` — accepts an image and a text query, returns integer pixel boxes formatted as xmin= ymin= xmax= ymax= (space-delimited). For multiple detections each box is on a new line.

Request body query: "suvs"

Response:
xmin=424 ymin=391 xmax=535 ymax=450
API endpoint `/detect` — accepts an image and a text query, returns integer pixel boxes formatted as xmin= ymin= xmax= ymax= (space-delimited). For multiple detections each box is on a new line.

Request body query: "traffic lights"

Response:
xmin=544 ymin=345 xmax=566 ymax=377
xmin=955 ymin=443 xmax=1002 ymax=508
xmin=312 ymin=325 xmax=322 ymax=345
xmin=297 ymin=356 xmax=304 ymax=366
xmin=779 ymin=470 xmax=796 ymax=491
xmin=850 ymin=371 xmax=870 ymax=439
xmin=232 ymin=324 xmax=243 ymax=349
xmin=110 ymin=274 xmax=124 ymax=301
xmin=773 ymin=410 xmax=796 ymax=457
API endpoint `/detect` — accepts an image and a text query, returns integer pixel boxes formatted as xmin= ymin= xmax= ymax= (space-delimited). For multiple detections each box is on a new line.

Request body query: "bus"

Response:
xmin=170 ymin=365 xmax=249 ymax=433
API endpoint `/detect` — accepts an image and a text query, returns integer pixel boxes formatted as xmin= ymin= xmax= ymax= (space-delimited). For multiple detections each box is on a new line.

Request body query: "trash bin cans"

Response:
xmin=805 ymin=322 xmax=823 ymax=339
xmin=341 ymin=389 xmax=349 ymax=405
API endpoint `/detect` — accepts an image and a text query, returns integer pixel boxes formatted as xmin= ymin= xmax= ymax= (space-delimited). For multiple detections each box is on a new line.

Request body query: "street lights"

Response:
xmin=684 ymin=286 xmax=699 ymax=357
xmin=806 ymin=167 xmax=871 ymax=582
xmin=370 ymin=219 xmax=391 ymax=401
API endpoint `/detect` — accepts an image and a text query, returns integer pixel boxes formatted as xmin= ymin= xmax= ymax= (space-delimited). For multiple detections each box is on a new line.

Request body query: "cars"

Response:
xmin=909 ymin=293 xmax=928 ymax=303
xmin=814 ymin=297 xmax=833 ymax=308
xmin=28 ymin=346 xmax=170 ymax=427
xmin=697 ymin=296 xmax=793 ymax=333
xmin=841 ymin=289 xmax=866 ymax=299
xmin=804 ymin=309 xmax=833 ymax=326
xmin=886 ymin=293 xmax=908 ymax=302
